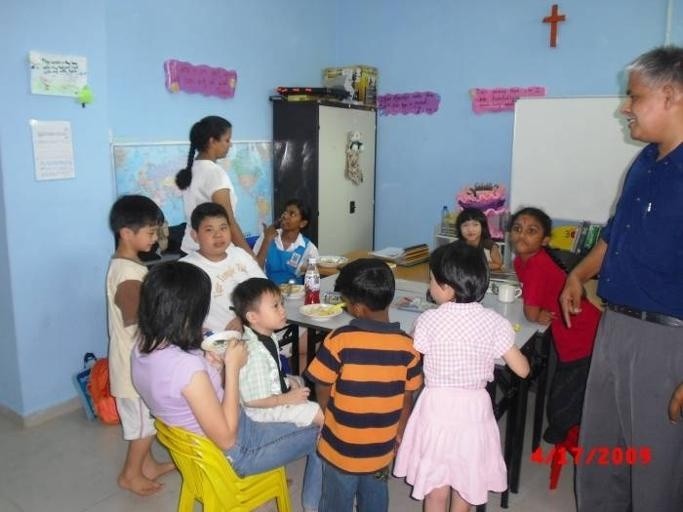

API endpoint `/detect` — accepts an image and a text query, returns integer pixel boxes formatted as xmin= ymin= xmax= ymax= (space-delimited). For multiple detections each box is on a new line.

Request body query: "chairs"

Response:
xmin=150 ymin=409 xmax=292 ymax=510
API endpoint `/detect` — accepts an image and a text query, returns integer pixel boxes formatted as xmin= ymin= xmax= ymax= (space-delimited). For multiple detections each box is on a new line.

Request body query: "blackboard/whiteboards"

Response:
xmin=508 ymin=96 xmax=651 ymax=227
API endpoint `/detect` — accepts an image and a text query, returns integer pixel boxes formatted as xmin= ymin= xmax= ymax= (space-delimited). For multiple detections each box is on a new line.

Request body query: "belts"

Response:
xmin=609 ymin=303 xmax=680 ymax=327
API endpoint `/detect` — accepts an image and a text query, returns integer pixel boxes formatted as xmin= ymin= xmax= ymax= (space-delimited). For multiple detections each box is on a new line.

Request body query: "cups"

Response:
xmin=497 ymin=282 xmax=522 ymax=302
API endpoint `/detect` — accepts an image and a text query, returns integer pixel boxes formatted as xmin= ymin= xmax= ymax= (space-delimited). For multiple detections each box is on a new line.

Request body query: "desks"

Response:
xmin=272 ymin=232 xmax=556 ymax=509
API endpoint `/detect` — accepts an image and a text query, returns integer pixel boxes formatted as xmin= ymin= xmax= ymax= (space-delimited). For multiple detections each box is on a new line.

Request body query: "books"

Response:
xmin=367 ymin=244 xmax=429 ymax=267
xmin=570 ymin=221 xmax=602 ymax=257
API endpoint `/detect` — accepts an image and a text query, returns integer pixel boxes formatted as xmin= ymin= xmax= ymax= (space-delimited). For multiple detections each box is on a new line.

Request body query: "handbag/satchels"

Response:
xmin=75 ymin=353 xmax=120 ymax=425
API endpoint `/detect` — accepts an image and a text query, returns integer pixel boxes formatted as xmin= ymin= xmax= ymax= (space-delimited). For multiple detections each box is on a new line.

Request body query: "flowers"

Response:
xmin=458 ymin=183 xmax=509 ymax=213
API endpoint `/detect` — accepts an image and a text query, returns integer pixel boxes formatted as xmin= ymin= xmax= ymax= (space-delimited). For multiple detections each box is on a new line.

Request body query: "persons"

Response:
xmin=508 ymin=208 xmax=603 ymax=444
xmin=176 ymin=116 xmax=255 ymax=257
xmin=254 ymin=199 xmax=318 ymax=286
xmin=302 ymin=257 xmax=425 ymax=511
xmin=393 ymin=243 xmax=529 ymax=510
xmin=454 ymin=207 xmax=500 ymax=270
xmin=105 ymin=196 xmax=177 ymax=496
xmin=558 ymin=45 xmax=682 ymax=508
xmin=229 ymin=279 xmax=326 ymax=448
xmin=177 ymin=203 xmax=321 ymax=376
xmin=130 ymin=259 xmax=324 ymax=511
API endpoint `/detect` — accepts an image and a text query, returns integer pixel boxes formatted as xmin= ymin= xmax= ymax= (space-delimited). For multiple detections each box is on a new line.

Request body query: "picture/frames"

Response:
xmin=110 ymin=139 xmax=272 ymax=254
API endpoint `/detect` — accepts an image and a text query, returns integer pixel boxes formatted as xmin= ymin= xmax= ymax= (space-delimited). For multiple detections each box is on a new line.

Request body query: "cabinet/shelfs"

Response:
xmin=269 ymin=96 xmax=378 ymax=277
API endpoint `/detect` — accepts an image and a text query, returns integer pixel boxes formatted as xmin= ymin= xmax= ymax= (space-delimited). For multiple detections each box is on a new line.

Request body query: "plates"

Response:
xmin=199 ymin=329 xmax=242 ymax=352
xmin=312 ymin=254 xmax=347 ymax=269
xmin=397 ymin=298 xmax=436 ymax=314
xmin=297 ymin=302 xmax=344 ymax=325
xmin=277 ymin=280 xmax=306 ymax=301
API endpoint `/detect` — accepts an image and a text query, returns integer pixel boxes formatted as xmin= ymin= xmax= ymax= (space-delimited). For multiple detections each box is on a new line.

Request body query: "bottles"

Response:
xmin=302 ymin=257 xmax=321 ymax=304
xmin=439 ymin=205 xmax=450 ymax=235
